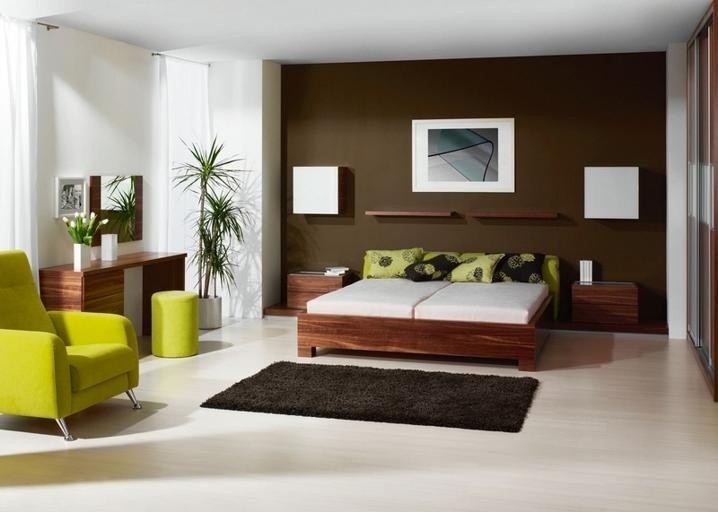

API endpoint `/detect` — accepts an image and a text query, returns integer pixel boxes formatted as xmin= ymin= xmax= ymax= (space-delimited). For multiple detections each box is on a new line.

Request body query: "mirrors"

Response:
xmin=90 ymin=176 xmax=143 ymax=245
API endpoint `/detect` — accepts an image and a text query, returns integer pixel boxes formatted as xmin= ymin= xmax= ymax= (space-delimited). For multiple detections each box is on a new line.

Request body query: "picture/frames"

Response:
xmin=411 ymin=118 xmax=516 ymax=193
xmin=55 ymin=177 xmax=88 ymax=218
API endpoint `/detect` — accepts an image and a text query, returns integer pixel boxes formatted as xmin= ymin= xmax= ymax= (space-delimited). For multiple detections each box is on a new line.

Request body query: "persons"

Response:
xmin=61 ymin=184 xmax=80 ymax=211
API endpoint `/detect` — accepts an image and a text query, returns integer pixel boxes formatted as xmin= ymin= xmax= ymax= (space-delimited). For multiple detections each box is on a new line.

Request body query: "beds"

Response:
xmin=297 ymin=254 xmax=561 ymax=370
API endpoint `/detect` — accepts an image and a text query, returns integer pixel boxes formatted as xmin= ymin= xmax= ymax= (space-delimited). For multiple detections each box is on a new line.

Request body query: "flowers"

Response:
xmin=63 ymin=212 xmax=109 ymax=243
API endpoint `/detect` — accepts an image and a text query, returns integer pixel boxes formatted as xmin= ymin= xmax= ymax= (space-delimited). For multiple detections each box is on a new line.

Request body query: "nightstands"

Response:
xmin=571 ymin=282 xmax=640 ymax=333
xmin=286 ymin=271 xmax=350 ymax=310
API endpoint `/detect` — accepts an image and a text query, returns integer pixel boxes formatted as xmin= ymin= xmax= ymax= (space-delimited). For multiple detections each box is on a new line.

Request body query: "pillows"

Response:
xmin=367 ymin=248 xmax=545 ymax=284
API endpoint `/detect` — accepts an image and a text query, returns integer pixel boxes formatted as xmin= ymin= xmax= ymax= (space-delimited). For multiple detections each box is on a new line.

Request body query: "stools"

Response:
xmin=150 ymin=289 xmax=200 ymax=358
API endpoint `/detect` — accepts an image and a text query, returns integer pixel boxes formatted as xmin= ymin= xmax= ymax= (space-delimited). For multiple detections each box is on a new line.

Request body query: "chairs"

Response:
xmin=0 ymin=249 xmax=142 ymax=441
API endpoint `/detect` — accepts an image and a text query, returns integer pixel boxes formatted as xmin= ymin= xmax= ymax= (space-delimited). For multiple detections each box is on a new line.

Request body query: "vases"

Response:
xmin=74 ymin=244 xmax=91 ymax=272
xmin=101 ymin=233 xmax=117 ymax=261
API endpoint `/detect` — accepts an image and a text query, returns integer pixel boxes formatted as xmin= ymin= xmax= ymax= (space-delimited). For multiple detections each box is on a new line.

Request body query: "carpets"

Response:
xmin=200 ymin=360 xmax=539 ymax=433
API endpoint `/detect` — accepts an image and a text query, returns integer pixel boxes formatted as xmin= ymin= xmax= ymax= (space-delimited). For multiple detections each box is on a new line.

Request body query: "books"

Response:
xmin=323 ymin=265 xmax=349 ymax=277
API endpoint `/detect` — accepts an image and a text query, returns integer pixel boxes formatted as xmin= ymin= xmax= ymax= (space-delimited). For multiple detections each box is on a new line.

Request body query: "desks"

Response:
xmin=39 ymin=251 xmax=187 ymax=337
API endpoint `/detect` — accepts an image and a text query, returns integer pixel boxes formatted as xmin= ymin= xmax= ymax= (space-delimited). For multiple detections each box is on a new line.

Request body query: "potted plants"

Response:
xmin=171 ymin=135 xmax=245 ymax=330
